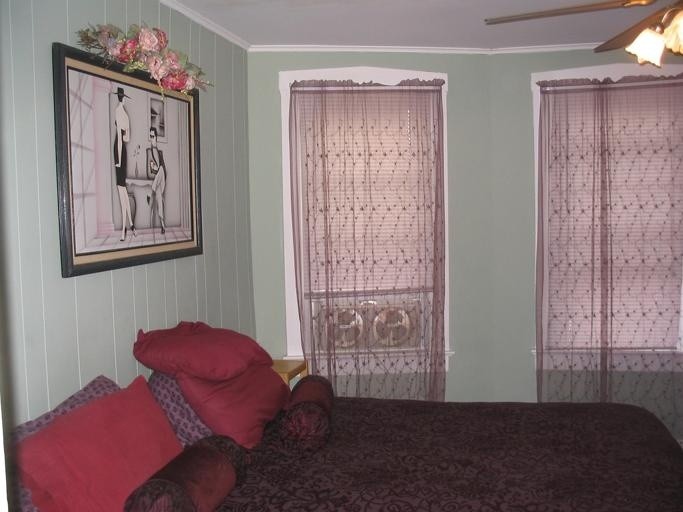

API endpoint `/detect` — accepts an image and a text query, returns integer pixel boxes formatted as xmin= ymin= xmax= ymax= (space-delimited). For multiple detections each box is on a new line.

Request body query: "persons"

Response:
xmin=145 ymin=127 xmax=166 ymax=235
xmin=112 ymin=86 xmax=137 ymax=243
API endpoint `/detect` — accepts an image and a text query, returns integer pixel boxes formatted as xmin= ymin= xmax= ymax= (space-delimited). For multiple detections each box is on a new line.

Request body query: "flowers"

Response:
xmin=74 ymin=17 xmax=214 ymax=104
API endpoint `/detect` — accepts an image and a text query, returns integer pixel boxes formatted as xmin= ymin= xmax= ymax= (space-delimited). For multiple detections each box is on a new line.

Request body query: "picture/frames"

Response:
xmin=50 ymin=38 xmax=204 ymax=280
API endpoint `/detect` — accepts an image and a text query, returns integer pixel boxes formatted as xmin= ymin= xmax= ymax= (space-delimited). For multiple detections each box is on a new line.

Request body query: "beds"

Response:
xmin=3 ymin=384 xmax=680 ymax=511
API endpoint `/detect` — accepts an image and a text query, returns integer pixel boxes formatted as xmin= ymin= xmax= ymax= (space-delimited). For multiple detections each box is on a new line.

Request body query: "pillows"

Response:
xmin=280 ymin=374 xmax=335 ymax=452
xmin=177 ymin=368 xmax=292 ymax=449
xmin=147 ymin=369 xmax=214 ymax=447
xmin=132 ymin=321 xmax=274 ymax=382
xmin=4 ymin=374 xmax=120 ymax=511
xmin=124 ymin=434 xmax=250 ymax=510
xmin=12 ymin=374 xmax=184 ymax=511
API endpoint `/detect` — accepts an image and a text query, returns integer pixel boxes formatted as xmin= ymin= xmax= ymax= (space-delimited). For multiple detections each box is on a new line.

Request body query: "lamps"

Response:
xmin=624 ymin=8 xmax=681 ymax=70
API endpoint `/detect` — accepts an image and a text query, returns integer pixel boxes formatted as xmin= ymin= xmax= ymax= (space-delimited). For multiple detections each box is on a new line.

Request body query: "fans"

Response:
xmin=482 ymin=0 xmax=682 ymax=55
